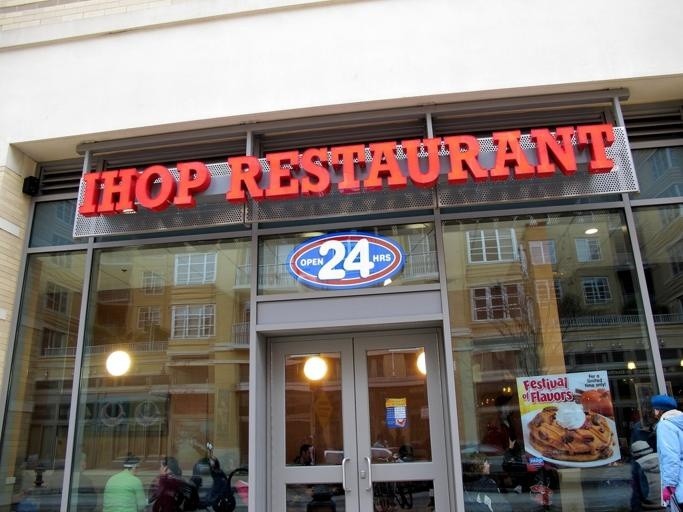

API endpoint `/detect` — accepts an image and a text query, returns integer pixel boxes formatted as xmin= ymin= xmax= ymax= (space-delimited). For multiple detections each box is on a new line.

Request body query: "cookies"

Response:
xmin=527 ymin=402 xmax=615 ymax=462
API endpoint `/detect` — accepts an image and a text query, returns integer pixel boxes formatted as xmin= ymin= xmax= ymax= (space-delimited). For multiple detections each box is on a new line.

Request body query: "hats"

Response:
xmin=651 ymin=394 xmax=677 ymax=410
xmin=630 ymin=440 xmax=653 ymax=457
xmin=123 ymin=458 xmax=140 ymax=467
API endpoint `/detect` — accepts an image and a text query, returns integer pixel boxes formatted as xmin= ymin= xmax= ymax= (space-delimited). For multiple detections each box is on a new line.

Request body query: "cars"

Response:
xmin=461 ymin=439 xmax=562 ymax=491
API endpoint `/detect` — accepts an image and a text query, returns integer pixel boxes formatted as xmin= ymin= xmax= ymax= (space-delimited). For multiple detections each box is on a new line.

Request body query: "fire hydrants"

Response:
xmin=33 ymin=463 xmax=46 ymax=486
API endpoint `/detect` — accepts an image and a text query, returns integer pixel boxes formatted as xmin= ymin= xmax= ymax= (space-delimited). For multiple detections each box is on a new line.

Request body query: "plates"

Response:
xmin=520 ymin=404 xmax=622 ymax=467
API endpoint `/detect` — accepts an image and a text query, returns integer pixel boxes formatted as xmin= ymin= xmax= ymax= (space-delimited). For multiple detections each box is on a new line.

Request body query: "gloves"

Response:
xmin=663 ymin=487 xmax=675 ymax=502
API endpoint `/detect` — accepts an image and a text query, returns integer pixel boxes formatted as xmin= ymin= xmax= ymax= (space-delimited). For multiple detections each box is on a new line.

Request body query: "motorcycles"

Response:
xmin=153 ymin=442 xmax=236 ymax=512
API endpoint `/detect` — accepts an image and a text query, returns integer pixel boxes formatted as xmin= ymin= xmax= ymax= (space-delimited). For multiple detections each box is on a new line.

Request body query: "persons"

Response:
xmin=292 ymin=444 xmax=328 ymax=491
xmin=464 ymin=452 xmax=513 ymax=512
xmin=58 ymin=450 xmax=97 ymax=512
xmin=650 ymin=394 xmax=683 ymax=512
xmin=103 ymin=453 xmax=185 ymax=512
xmin=630 ymin=440 xmax=665 ymax=512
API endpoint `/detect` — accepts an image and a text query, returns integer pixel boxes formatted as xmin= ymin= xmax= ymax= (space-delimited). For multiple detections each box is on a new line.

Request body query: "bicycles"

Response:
xmin=373 ymin=450 xmax=413 ymax=512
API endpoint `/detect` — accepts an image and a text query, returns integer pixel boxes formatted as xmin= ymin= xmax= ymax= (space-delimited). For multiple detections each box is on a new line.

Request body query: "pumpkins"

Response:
xmin=580 ymin=388 xmax=614 ymax=417
